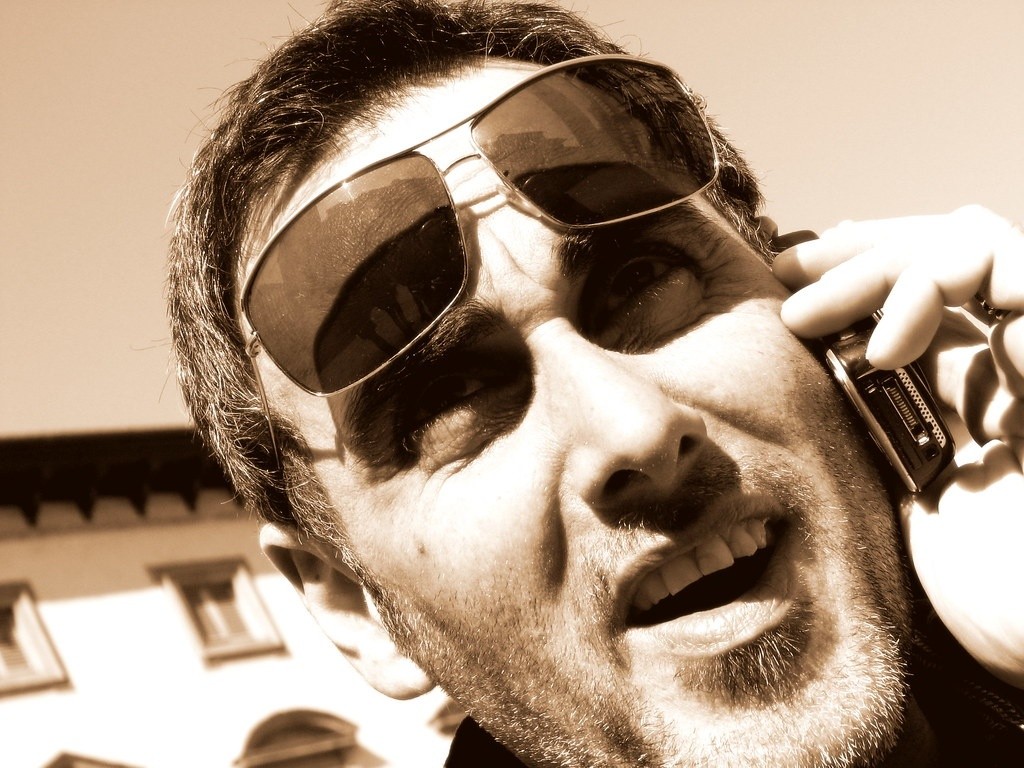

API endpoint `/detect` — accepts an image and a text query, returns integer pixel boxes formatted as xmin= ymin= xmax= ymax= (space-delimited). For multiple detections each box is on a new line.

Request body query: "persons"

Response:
xmin=167 ymin=0 xmax=1024 ymax=768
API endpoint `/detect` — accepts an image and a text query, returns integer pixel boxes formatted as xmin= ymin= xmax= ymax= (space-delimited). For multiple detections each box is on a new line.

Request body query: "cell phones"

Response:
xmin=771 ymin=230 xmax=957 ymax=494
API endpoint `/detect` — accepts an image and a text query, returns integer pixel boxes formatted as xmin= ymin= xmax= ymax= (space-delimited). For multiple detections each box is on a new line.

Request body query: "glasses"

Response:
xmin=234 ymin=53 xmax=762 ymax=523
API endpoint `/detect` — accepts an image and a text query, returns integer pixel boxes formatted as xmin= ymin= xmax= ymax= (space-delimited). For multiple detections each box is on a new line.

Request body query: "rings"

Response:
xmin=974 ymin=292 xmax=1001 ymax=315
